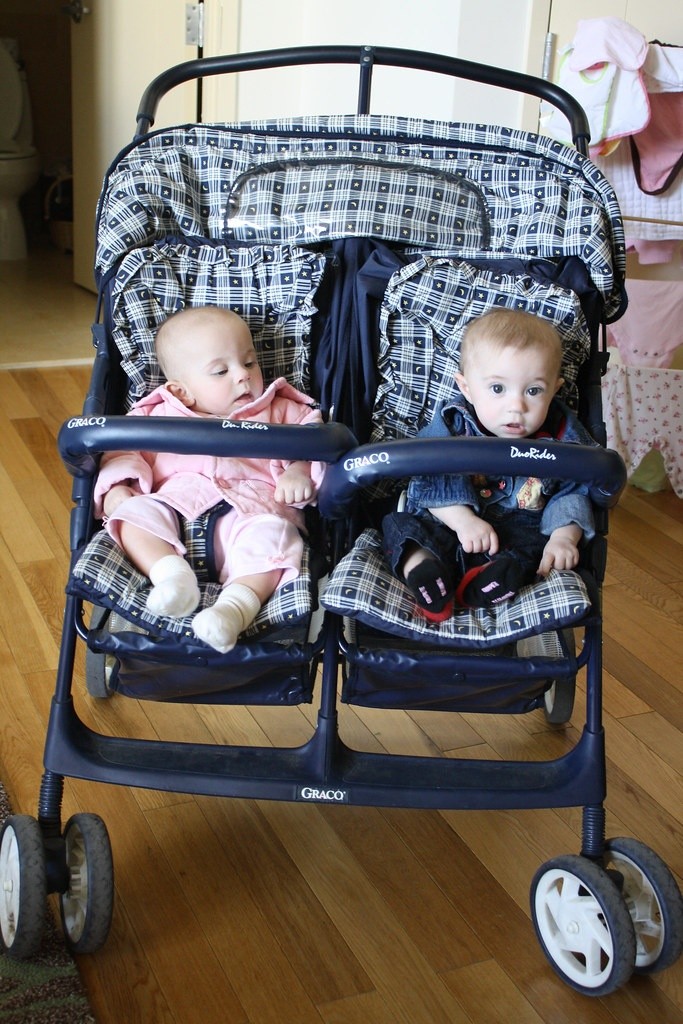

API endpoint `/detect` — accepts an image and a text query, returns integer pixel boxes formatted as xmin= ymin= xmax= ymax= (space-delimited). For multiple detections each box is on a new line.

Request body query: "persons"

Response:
xmin=380 ymin=307 xmax=599 ymax=622
xmin=93 ymin=306 xmax=329 ymax=655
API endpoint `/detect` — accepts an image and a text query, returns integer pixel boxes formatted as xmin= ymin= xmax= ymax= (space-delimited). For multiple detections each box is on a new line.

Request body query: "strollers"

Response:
xmin=0 ymin=44 xmax=683 ymax=1000
